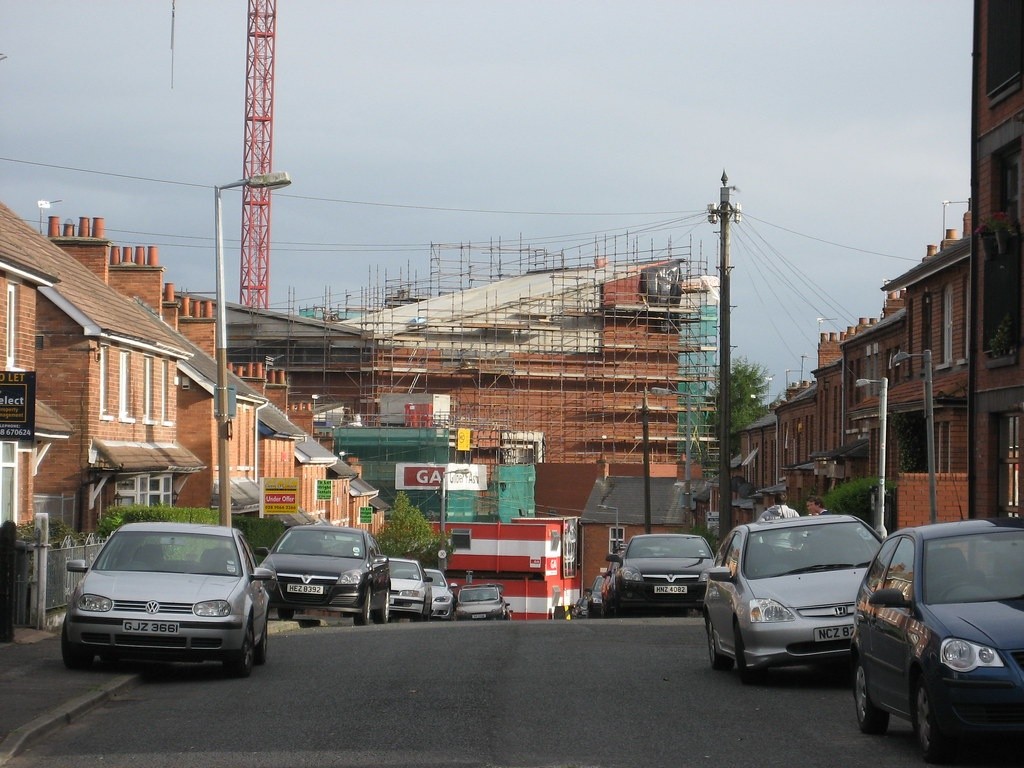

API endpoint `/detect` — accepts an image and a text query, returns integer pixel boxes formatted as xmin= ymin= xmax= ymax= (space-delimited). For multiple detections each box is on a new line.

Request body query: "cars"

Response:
xmin=60 ymin=521 xmax=275 ymax=679
xmin=571 ymin=545 xmax=628 ymax=622
xmin=254 ymin=524 xmax=392 ymax=626
xmin=411 ymin=568 xmax=458 ymax=622
xmin=849 ymin=521 xmax=1024 ymax=766
xmin=386 ymin=557 xmax=434 ymax=622
xmin=606 ymin=533 xmax=716 ymax=619
xmin=456 ymin=583 xmax=513 ymax=621
xmin=703 ymin=514 xmax=910 ymax=687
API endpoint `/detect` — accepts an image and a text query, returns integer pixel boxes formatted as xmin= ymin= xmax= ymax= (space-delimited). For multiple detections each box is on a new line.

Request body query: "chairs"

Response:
xmin=928 ymin=549 xmax=972 ymax=597
xmin=135 ymin=545 xmax=163 ymax=564
xmin=198 ymin=547 xmax=232 ymax=566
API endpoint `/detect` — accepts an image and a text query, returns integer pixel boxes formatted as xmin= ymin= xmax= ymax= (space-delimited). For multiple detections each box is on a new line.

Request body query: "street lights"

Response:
xmin=892 ymin=349 xmax=937 ymax=525
xmin=856 ymin=376 xmax=888 ymax=541
xmin=651 ymin=387 xmax=692 ymax=535
xmin=214 ymin=171 xmax=293 ymax=526
xmin=39 ymin=198 xmax=61 ymax=235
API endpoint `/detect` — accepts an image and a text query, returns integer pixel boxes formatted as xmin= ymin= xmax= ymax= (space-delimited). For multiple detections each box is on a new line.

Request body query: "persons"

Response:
xmin=807 ymin=496 xmax=832 ymax=516
xmin=756 ymin=492 xmax=807 ymax=551
